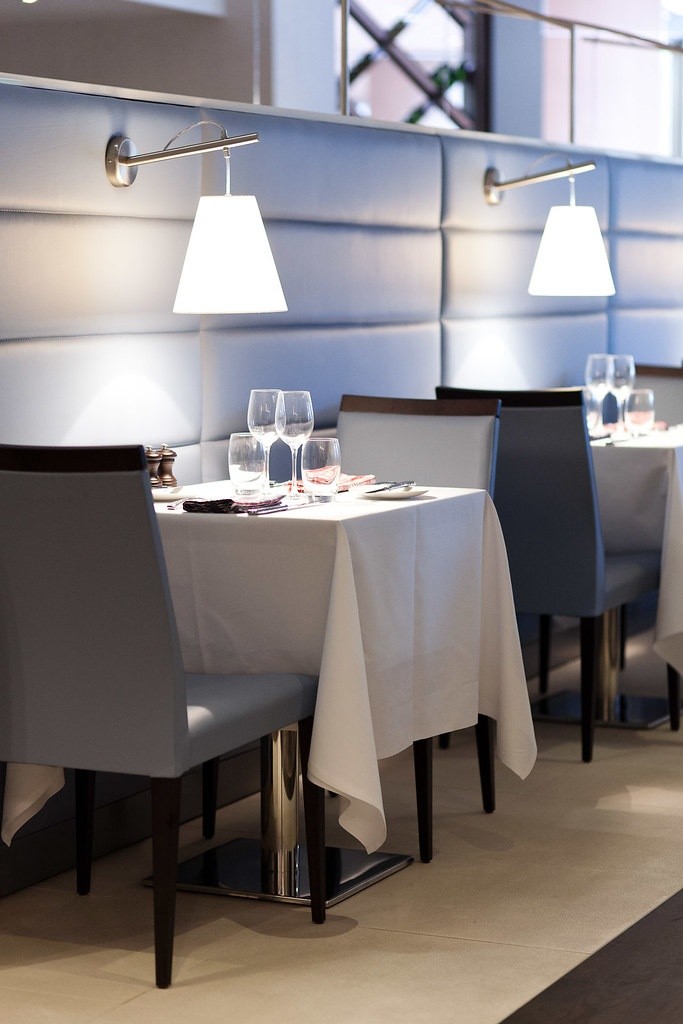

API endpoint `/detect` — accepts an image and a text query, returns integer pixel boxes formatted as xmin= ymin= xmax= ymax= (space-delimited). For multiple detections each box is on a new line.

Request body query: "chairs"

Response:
xmin=326 ymin=394 xmax=496 ymax=864
xmin=436 ymin=381 xmax=678 ymax=763
xmin=0 ymin=442 xmax=325 ymax=984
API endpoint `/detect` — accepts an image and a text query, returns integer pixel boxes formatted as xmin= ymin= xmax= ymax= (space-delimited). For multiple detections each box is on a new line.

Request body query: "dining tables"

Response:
xmin=142 ymin=472 xmax=539 ymax=909
xmin=592 ymin=424 xmax=682 ymax=674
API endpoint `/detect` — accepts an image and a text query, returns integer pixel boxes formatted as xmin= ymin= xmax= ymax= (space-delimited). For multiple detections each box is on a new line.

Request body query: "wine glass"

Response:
xmin=247 ymin=389 xmax=285 ymax=498
xmin=275 ymin=391 xmax=314 ymax=498
xmin=585 ymin=354 xmax=613 ymax=439
xmin=607 ymin=355 xmax=635 ymax=440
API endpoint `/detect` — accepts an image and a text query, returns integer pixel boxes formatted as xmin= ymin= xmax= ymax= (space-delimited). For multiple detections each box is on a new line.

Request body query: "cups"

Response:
xmin=625 ymin=389 xmax=654 ymax=436
xmin=228 ymin=433 xmax=267 ymax=496
xmin=301 ymin=438 xmax=341 ymax=502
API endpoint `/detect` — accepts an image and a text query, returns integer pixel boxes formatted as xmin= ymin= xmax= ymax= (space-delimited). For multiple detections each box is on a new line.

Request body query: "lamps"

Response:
xmin=105 ymin=121 xmax=288 ymax=315
xmin=483 ymin=154 xmax=616 ymax=297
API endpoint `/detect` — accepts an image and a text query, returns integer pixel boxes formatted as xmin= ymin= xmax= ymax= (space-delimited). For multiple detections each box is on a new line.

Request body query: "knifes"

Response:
xmin=365 ymin=481 xmax=415 ymax=493
xmin=247 ymin=502 xmax=308 ymax=515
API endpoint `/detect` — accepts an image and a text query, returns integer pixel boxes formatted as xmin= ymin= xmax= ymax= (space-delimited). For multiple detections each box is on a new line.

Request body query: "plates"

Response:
xmin=349 ymin=484 xmax=428 ymax=498
